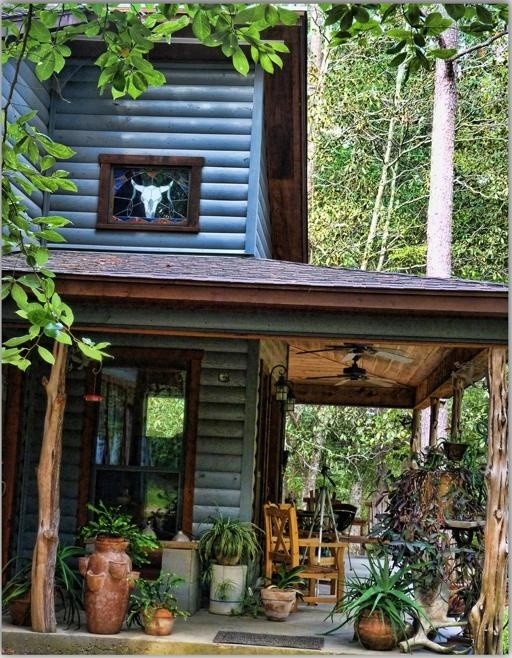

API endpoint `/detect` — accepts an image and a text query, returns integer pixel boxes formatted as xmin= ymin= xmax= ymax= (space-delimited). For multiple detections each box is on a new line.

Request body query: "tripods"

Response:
xmin=301 ymin=486 xmax=352 ymax=607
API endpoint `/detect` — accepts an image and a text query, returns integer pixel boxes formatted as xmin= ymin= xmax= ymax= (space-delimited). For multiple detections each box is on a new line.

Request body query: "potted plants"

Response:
xmin=318 ymin=436 xmax=487 ymax=652
xmin=195 ymin=504 xmax=264 ymax=568
xmin=252 ymin=561 xmax=306 ymax=621
xmin=1 ymin=500 xmax=188 ymax=637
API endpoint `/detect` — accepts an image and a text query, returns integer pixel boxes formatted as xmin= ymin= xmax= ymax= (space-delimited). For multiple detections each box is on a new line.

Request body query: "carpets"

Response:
xmin=212 ymin=630 xmax=324 ymax=651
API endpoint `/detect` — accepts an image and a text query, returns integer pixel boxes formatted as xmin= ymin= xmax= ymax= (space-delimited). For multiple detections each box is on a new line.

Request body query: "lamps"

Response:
xmin=269 ymin=365 xmax=297 ymax=412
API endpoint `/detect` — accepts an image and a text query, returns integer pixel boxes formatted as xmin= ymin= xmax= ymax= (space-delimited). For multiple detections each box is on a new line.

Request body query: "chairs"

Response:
xmin=264 ymin=502 xmax=347 ymax=615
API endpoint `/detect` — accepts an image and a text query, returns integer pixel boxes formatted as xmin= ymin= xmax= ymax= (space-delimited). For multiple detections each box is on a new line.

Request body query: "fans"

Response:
xmin=295 ymin=342 xmax=416 ymax=388
